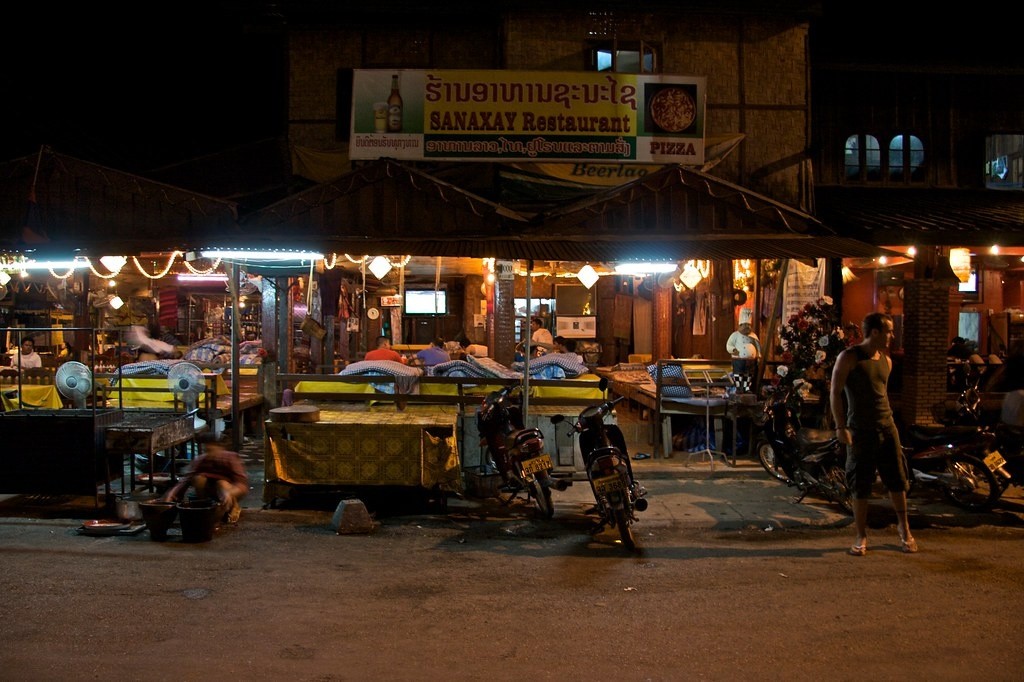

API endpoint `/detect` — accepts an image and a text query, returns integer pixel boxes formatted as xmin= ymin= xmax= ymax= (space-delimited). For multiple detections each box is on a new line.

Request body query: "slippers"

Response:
xmin=632 ymin=451 xmax=650 ymax=459
xmin=897 ymin=524 xmax=917 ymax=554
xmin=227 ymin=506 xmax=242 ymax=523
xmin=849 ymin=544 xmax=867 ymax=556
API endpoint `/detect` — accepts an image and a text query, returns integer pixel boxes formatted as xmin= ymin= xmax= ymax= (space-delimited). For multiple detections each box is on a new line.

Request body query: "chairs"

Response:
xmin=0 ymin=364 xmax=57 ymax=385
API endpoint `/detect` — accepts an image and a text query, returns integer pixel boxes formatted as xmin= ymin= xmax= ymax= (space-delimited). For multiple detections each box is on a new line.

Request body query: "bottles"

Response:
xmin=289 ymin=345 xmax=350 ymax=374
xmin=1002 ymin=306 xmax=1024 ymax=354
xmin=399 ymin=351 xmax=411 ymax=364
xmin=98 ymin=340 xmax=103 ymax=355
xmin=178 ymin=296 xmax=263 ymax=343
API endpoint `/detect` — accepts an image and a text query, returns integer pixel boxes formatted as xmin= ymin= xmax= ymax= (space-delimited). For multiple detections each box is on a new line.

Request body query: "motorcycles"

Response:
xmin=755 ymin=376 xmax=872 ymax=515
xmin=551 ymin=377 xmax=647 ymax=549
xmin=835 ymin=393 xmax=1011 ymax=511
xmin=931 ymin=364 xmax=1024 ymax=490
xmin=475 ymin=381 xmax=574 ymax=519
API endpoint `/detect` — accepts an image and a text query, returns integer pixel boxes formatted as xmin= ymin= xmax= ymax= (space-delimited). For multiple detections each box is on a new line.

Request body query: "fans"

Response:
xmin=55 ymin=361 xmax=92 ymax=409
xmin=166 ymin=363 xmax=207 ymax=429
xmin=87 ymin=290 xmax=115 ymax=308
xmin=226 ymin=276 xmax=264 ymax=295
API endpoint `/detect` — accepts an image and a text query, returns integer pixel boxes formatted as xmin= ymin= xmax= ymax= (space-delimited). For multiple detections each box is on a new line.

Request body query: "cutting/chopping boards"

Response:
xmin=269 ymin=406 xmax=320 ymax=422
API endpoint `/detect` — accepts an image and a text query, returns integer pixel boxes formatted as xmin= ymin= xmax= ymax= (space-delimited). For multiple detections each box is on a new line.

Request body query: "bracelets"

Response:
xmin=536 ymin=342 xmax=542 ymax=347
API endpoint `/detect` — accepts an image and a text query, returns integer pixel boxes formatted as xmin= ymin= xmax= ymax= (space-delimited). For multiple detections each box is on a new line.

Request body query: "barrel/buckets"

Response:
xmin=138 ymin=501 xmax=177 ymax=541
xmin=176 ymin=502 xmax=217 ymax=543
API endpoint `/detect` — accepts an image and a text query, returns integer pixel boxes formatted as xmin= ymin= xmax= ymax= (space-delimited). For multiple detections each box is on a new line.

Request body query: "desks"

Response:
xmin=86 ymin=369 xmax=280 ymax=452
xmin=0 ymin=381 xmax=64 ymax=412
xmin=165 ymin=429 xmax=204 ymax=460
xmin=265 ymin=344 xmax=617 ymax=516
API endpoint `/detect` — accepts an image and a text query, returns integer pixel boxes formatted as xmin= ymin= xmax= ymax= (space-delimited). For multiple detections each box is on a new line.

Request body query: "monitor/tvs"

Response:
xmin=405 ymin=288 xmax=446 ymax=315
xmin=957 ymin=267 xmax=979 ymax=293
xmin=554 ymin=284 xmax=597 ymax=316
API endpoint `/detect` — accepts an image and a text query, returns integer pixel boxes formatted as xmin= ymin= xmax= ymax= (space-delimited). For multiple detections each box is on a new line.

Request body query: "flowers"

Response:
xmin=769 ymin=294 xmax=859 ymax=394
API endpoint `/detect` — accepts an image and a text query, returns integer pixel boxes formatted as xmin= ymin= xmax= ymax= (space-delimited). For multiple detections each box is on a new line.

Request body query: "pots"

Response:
xmin=114 ymin=494 xmax=148 ymax=521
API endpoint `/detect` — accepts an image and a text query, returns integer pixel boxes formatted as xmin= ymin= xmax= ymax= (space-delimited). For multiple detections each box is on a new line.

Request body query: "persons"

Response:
xmin=947 ymin=336 xmax=973 ymax=360
xmin=364 ymin=336 xmax=408 ymax=365
xmin=829 ymin=312 xmax=917 ymax=556
xmin=11 ymin=336 xmax=42 ymax=368
xmin=998 ymin=342 xmax=1008 ymax=360
xmin=726 ymin=308 xmax=761 ymax=382
xmin=406 ymin=335 xmax=452 ymax=365
xmin=166 ymin=431 xmax=249 ymax=524
xmin=515 ymin=318 xmax=576 ymax=360
xmin=58 ymin=341 xmax=72 ymax=357
xmin=459 ymin=337 xmax=488 ymax=357
xmin=193 ymin=326 xmax=204 ymax=344
xmin=225 ymin=323 xmax=231 ymax=335
xmin=158 ymin=325 xmax=180 ymax=345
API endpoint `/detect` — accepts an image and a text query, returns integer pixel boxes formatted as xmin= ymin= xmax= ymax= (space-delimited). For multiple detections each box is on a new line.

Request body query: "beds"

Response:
xmin=590 ymin=365 xmax=736 ymax=458
